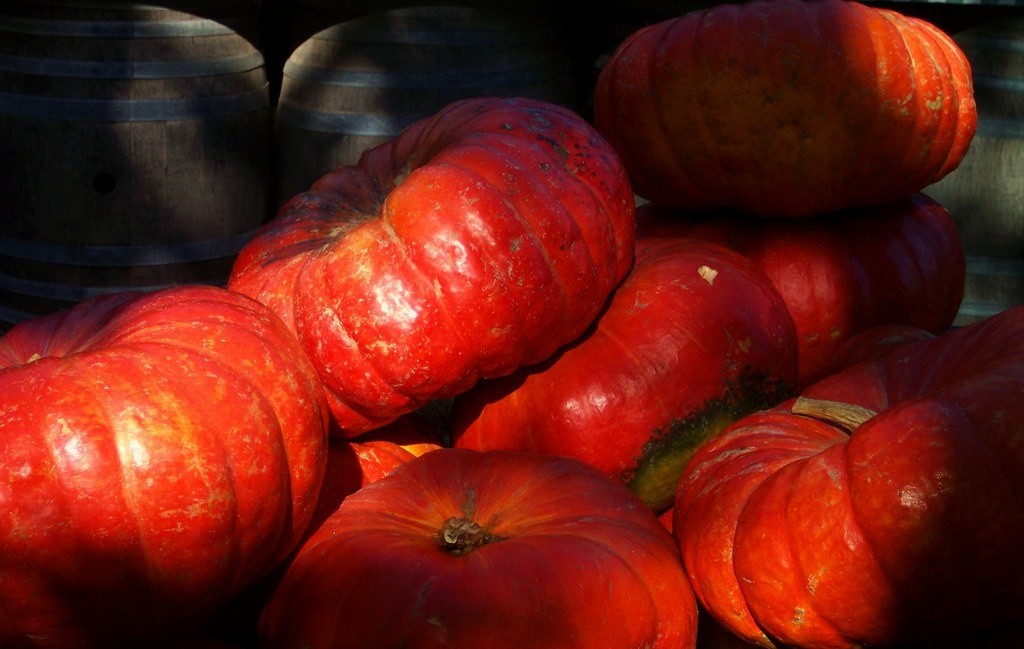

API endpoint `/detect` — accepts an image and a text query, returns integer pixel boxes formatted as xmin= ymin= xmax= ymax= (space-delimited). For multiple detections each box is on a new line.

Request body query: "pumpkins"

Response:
xmin=1 ymin=0 xmax=1024 ymax=649
